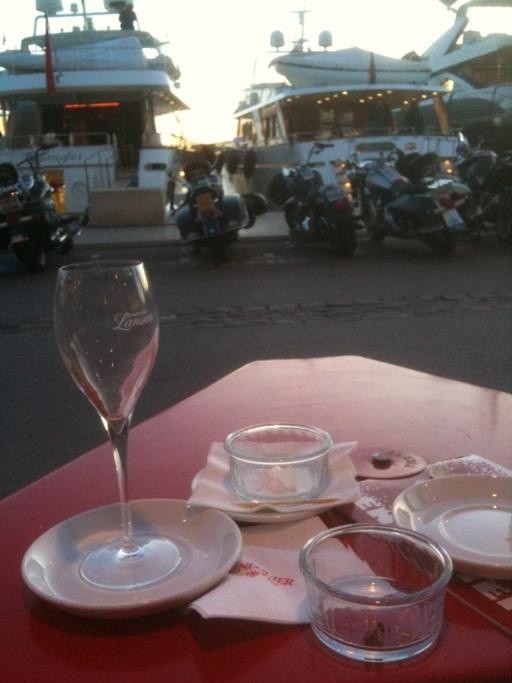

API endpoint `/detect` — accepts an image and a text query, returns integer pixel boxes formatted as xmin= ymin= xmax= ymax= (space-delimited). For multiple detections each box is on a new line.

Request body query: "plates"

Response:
xmin=188 ymin=465 xmax=338 ymax=527
xmin=389 ymin=470 xmax=512 ymax=584
xmin=18 ymin=495 xmax=245 ymax=623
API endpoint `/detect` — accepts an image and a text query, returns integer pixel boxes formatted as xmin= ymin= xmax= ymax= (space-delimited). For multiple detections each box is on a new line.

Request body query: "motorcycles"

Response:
xmin=0 ymin=143 xmax=87 ymax=270
xmin=448 ymin=132 xmax=511 ymax=246
xmin=268 ymin=141 xmax=357 ymax=261
xmin=140 ymin=147 xmax=268 ymax=267
xmin=334 ymin=147 xmax=480 ymax=252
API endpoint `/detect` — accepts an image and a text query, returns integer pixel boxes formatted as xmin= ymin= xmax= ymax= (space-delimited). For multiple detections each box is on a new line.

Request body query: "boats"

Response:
xmin=392 ymin=0 xmax=512 ymax=138
xmin=232 ymin=9 xmax=467 ymax=205
xmin=0 ymin=0 xmax=188 ymax=215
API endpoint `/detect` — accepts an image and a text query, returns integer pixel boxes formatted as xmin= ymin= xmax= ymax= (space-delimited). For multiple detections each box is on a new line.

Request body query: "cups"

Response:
xmin=299 ymin=519 xmax=455 ymax=665
xmin=220 ymin=419 xmax=331 ymax=502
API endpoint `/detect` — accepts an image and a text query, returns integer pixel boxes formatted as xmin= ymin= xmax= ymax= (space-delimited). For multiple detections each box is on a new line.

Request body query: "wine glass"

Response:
xmin=51 ymin=257 xmax=184 ymax=591
